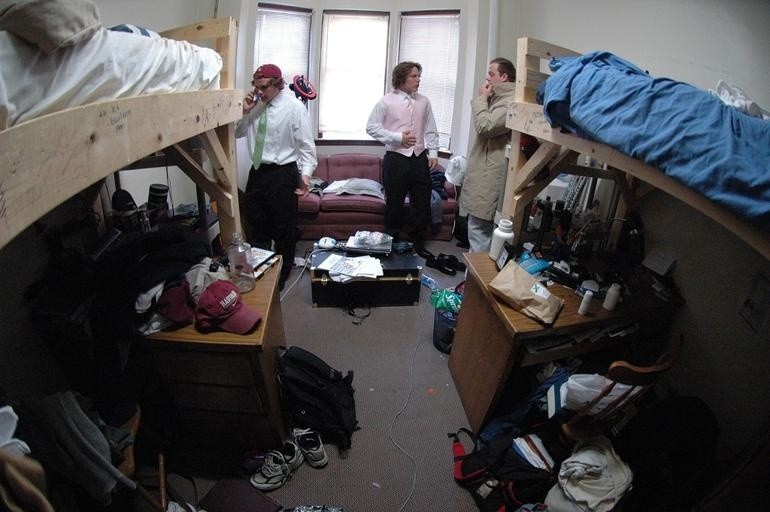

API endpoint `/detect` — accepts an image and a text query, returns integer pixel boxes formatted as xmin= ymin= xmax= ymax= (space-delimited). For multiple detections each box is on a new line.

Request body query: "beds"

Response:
xmin=0 ymin=15 xmax=245 ymax=253
xmin=500 ymin=36 xmax=770 ymax=261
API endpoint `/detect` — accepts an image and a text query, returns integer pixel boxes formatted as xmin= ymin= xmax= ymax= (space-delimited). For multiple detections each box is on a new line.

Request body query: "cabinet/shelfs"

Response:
xmin=146 ymin=254 xmax=287 ymax=457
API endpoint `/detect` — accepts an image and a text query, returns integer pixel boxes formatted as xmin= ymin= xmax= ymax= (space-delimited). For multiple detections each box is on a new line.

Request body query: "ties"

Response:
xmin=253 ymin=102 xmax=269 ymax=171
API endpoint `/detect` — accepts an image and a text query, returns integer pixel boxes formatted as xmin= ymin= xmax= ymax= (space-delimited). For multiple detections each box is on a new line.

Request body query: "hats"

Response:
xmin=253 ymin=65 xmax=287 ymax=86
xmin=195 ymin=281 xmax=261 ymax=335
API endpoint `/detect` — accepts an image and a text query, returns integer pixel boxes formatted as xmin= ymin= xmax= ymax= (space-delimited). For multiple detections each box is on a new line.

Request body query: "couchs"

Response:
xmin=297 ymin=153 xmax=457 ymax=240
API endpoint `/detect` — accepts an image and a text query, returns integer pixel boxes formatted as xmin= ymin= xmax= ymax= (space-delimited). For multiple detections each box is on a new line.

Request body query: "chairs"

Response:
xmin=524 ymin=334 xmax=685 ymax=434
xmin=38 ymin=389 xmax=169 ymax=512
xmin=132 ymin=472 xmax=282 ymax=512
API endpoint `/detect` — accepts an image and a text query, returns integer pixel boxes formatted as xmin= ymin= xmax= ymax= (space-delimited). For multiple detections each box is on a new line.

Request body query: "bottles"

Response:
xmin=227 ymin=233 xmax=256 ymax=293
xmin=421 ymin=275 xmax=438 ymax=289
xmin=603 ymin=283 xmax=621 ymax=311
xmin=543 ymin=196 xmax=553 ymax=208
xmin=430 ymin=287 xmax=462 ymax=313
xmin=489 ymin=219 xmax=514 ymax=262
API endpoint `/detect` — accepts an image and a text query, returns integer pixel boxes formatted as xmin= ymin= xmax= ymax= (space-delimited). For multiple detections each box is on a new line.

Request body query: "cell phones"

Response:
xmin=253 ymin=94 xmax=259 ymax=101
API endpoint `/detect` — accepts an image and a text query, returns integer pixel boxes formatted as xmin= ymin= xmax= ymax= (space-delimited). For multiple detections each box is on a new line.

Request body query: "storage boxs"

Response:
xmin=310 ymin=238 xmax=423 ymax=308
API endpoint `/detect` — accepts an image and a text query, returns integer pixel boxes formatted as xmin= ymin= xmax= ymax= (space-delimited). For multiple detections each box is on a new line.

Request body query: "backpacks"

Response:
xmin=272 ymin=340 xmax=355 ymax=452
xmin=453 ymin=417 xmax=560 ymax=512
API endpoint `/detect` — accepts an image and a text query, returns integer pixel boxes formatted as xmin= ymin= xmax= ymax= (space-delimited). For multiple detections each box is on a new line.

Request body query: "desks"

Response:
xmin=448 ymin=251 xmax=687 ymax=434
xmin=175 ymin=214 xmax=220 ymax=243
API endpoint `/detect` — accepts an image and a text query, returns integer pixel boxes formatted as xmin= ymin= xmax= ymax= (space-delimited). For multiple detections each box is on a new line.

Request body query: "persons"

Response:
xmin=236 ymin=65 xmax=319 ymax=292
xmin=459 ymin=58 xmax=518 ymax=283
xmin=367 ymin=61 xmax=439 ymax=259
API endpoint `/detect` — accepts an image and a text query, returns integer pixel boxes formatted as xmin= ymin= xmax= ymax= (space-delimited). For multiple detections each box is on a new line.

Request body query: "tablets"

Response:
xmin=227 ymin=246 xmax=276 ymax=272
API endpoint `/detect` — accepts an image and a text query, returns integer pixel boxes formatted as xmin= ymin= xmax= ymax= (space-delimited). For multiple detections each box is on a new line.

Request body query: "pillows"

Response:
xmin=336 ymin=178 xmax=383 ymax=199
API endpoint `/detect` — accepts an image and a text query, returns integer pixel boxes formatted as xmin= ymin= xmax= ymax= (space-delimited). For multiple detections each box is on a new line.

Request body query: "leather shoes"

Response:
xmin=439 ymin=254 xmax=466 ymax=270
xmin=417 ymin=248 xmax=434 ymax=259
xmin=427 ymin=257 xmax=455 ymax=275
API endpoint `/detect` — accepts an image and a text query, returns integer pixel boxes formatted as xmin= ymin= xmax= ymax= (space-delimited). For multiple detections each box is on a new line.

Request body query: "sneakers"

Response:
xmin=288 ymin=419 xmax=328 ymax=468
xmin=250 ymin=441 xmax=304 ymax=491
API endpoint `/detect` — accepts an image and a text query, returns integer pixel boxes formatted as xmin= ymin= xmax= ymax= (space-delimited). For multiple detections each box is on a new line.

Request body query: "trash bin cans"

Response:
xmin=433 ymin=304 xmax=458 ymax=353
